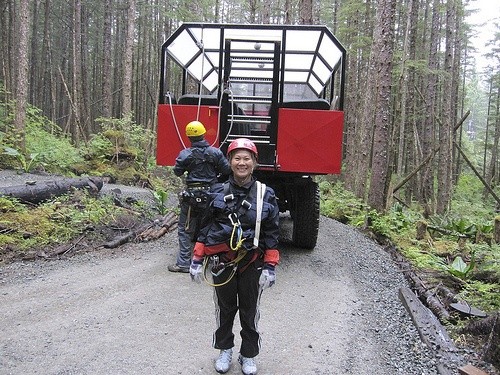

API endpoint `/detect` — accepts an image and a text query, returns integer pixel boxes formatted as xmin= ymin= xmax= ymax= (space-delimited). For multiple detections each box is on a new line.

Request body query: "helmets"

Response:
xmin=226 ymin=137 xmax=259 ymax=159
xmin=184 ymin=121 xmax=207 ymax=139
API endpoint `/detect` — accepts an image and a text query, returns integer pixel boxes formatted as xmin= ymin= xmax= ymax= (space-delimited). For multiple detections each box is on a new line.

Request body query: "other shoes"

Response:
xmin=168 ymin=263 xmax=192 ymax=273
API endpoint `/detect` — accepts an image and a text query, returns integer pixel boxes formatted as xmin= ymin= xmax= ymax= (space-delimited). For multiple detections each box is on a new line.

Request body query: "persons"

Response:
xmin=168 ymin=121 xmax=232 ymax=272
xmin=190 ymin=138 xmax=280 ymax=375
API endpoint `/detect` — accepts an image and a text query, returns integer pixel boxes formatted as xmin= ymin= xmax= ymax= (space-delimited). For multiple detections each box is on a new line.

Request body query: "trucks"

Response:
xmin=156 ymin=22 xmax=347 ymax=249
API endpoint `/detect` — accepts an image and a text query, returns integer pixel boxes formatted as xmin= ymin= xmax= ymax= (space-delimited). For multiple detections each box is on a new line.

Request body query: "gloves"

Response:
xmin=189 ymin=258 xmax=205 ymax=285
xmin=257 ymin=263 xmax=277 ymax=289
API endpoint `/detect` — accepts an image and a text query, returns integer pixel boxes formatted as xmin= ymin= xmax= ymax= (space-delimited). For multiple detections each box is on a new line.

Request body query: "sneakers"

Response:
xmin=237 ymin=354 xmax=257 ymax=375
xmin=212 ymin=348 xmax=233 ymax=375
